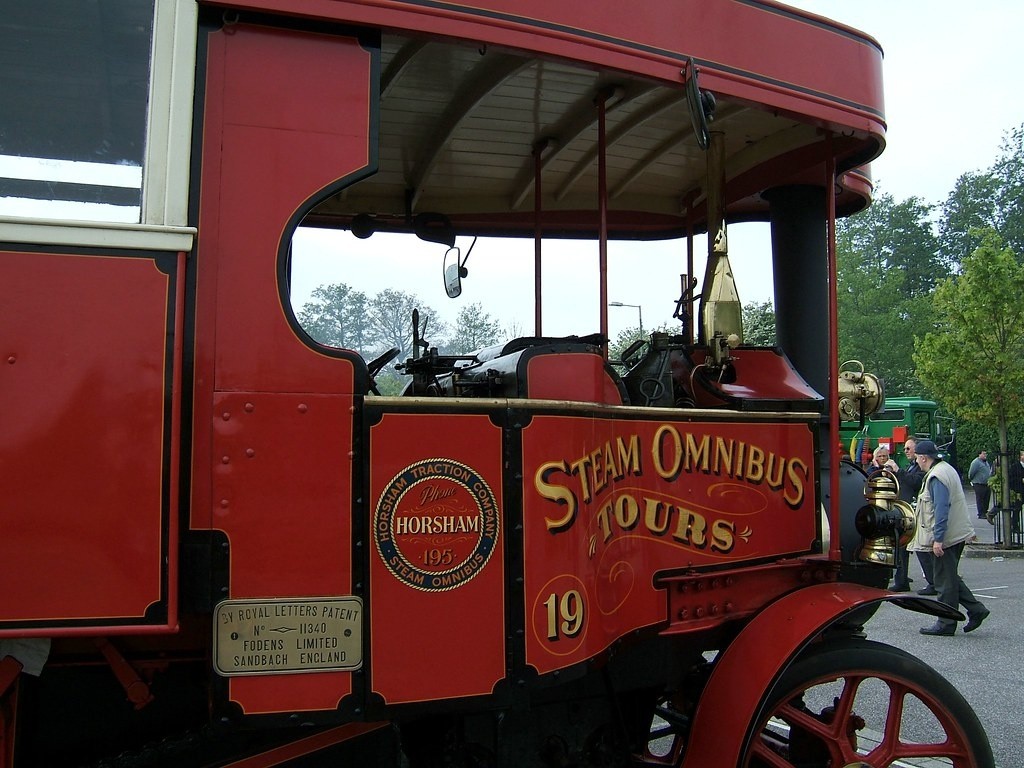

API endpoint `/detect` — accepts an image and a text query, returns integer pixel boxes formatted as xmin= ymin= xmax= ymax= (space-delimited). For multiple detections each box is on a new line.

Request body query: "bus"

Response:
xmin=838 ymin=395 xmax=957 ymax=502
xmin=1 ymin=0 xmax=1001 ymax=768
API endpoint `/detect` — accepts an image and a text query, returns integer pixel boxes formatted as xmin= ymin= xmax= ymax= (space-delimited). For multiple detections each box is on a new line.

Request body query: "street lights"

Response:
xmin=609 ymin=302 xmax=643 ymax=357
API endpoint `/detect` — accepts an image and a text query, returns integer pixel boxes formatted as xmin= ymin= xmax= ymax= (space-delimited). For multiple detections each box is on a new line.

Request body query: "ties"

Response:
xmin=906 ymin=462 xmax=914 ymax=471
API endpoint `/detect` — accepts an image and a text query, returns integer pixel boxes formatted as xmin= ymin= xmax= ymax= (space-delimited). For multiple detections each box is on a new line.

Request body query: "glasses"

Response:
xmin=903 ymin=446 xmax=915 ymax=451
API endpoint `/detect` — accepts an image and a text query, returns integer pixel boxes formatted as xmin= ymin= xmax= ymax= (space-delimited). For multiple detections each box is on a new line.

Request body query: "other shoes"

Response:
xmin=985 ymin=512 xmax=995 ymax=525
xmin=889 ymin=585 xmax=910 ymax=591
xmin=1013 ymin=529 xmax=1021 ymax=534
xmin=908 ymin=577 xmax=913 ymax=582
xmin=923 ymin=584 xmax=930 ymax=589
xmin=923 ymin=573 xmax=926 ymax=577
xmin=917 ymin=585 xmax=937 ymax=595
xmin=978 ymin=515 xmax=987 ymax=519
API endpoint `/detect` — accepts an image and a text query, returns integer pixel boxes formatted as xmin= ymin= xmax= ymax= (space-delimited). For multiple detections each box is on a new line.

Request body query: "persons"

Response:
xmin=985 ymin=444 xmax=1024 ymax=534
xmin=866 ymin=436 xmax=992 ymax=636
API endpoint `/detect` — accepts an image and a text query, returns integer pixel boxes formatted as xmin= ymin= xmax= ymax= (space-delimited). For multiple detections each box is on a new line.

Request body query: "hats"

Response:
xmin=914 ymin=441 xmax=946 ymax=459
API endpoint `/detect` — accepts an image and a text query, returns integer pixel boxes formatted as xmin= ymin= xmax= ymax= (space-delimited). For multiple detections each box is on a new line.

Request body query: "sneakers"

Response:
xmin=963 ymin=605 xmax=990 ymax=632
xmin=920 ymin=623 xmax=956 ymax=636
